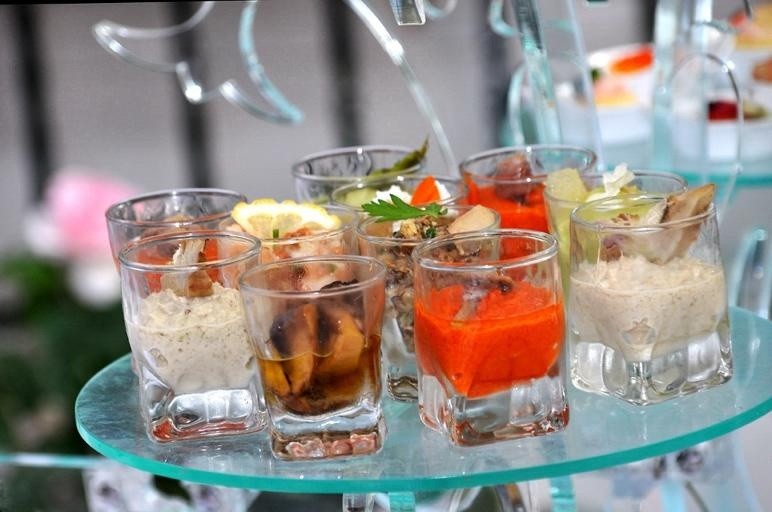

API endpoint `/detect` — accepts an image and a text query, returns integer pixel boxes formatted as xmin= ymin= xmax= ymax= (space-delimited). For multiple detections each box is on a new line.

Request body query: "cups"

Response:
xmin=237 ymin=256 xmax=388 ymax=464
xmin=459 ymin=149 xmax=598 ymax=233
xmin=559 ymin=194 xmax=732 ymax=404
xmin=325 ymin=175 xmax=466 ymax=217
xmin=579 ymin=170 xmax=687 ymax=203
xmin=410 ymin=230 xmax=568 ymax=442
xmin=99 ymin=188 xmax=252 ymax=266
xmin=218 ymin=204 xmax=361 ymax=270
xmin=118 ymin=239 xmax=264 ymax=443
xmin=289 ymin=146 xmax=427 ymax=206
xmin=352 ymin=203 xmax=502 ymax=402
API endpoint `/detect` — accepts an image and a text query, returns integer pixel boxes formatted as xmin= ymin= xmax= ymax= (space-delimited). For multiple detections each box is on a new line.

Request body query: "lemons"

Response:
xmin=229 ymin=200 xmax=341 ymax=241
